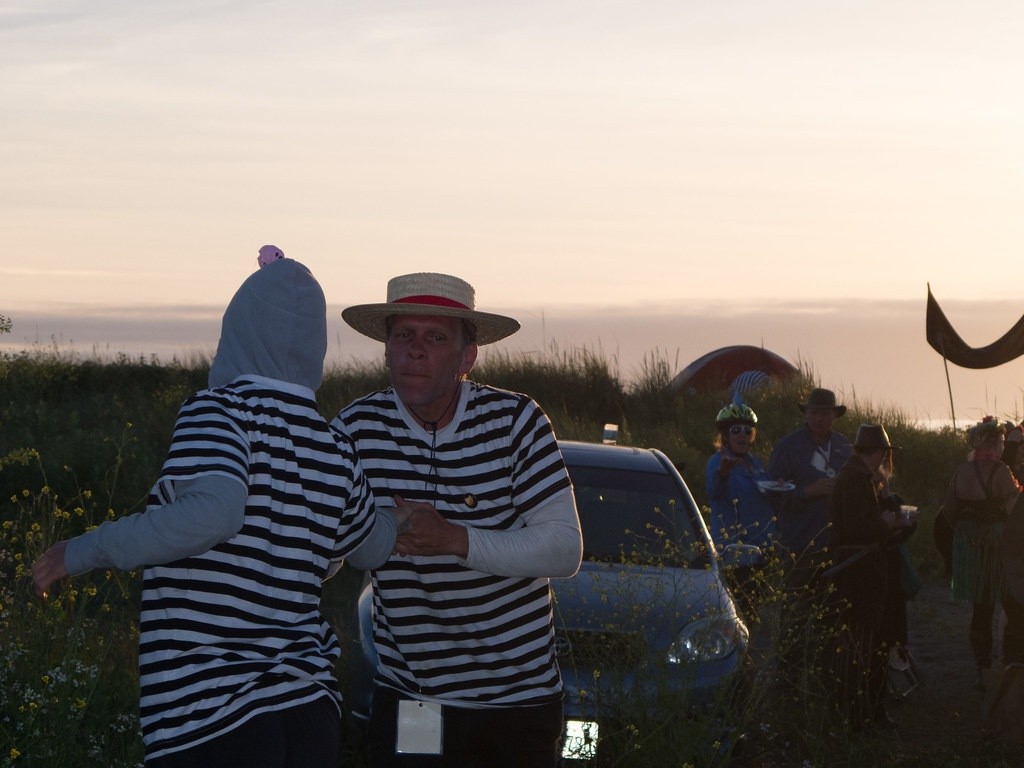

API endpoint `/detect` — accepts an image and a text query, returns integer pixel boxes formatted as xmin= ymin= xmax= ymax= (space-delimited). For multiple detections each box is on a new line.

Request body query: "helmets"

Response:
xmin=715 ymin=403 xmax=758 ymax=430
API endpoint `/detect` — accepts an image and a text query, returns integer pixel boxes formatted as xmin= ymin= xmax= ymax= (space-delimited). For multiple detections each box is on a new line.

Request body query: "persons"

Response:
xmin=825 ymin=424 xmax=898 ymax=732
xmin=767 ymin=387 xmax=854 ymax=692
xmin=1001 ymin=426 xmax=1024 ymax=486
xmin=993 ymin=487 xmax=1024 ymax=719
xmin=328 ymin=272 xmax=585 ymax=768
xmin=30 ymin=244 xmax=414 ymax=768
xmin=706 ymin=403 xmax=777 ymax=631
xmin=877 ymin=479 xmax=918 ymax=671
xmin=946 ymin=416 xmax=1024 ymax=688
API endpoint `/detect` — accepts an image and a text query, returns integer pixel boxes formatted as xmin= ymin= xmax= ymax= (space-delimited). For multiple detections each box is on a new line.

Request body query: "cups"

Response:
xmin=901 ymin=505 xmax=917 ymax=527
xmin=603 ymin=423 xmax=619 ymax=446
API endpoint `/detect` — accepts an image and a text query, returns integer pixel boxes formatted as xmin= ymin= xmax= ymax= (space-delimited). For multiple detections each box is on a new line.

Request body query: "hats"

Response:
xmin=844 ymin=424 xmax=902 ymax=450
xmin=797 ymin=388 xmax=847 ymax=418
xmin=342 ymin=273 xmax=521 ymax=347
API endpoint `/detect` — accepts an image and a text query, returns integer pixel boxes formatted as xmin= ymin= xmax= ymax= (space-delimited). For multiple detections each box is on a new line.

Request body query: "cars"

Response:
xmin=356 ymin=440 xmax=764 ymax=726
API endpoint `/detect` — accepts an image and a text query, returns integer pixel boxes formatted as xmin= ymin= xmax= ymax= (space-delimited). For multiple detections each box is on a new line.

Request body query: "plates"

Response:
xmin=758 ymin=481 xmax=797 ymax=491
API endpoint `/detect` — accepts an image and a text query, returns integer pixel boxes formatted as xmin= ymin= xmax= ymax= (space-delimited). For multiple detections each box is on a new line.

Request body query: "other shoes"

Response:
xmin=853 ymin=703 xmax=901 ymax=732
xmin=973 ymin=658 xmax=1024 ymax=697
